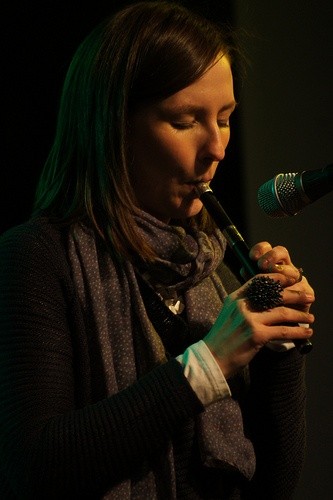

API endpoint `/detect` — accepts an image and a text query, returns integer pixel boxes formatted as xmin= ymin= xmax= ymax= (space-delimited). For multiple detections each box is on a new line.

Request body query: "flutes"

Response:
xmin=194 ymin=179 xmax=313 ymax=355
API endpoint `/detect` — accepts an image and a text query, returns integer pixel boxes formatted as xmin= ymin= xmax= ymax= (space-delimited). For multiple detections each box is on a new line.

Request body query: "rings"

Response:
xmin=247 ymin=275 xmax=282 ymax=309
xmin=296 ymin=268 xmax=303 ymax=282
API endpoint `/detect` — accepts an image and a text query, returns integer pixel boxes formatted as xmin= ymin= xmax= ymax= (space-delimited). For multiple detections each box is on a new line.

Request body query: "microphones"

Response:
xmin=258 ymin=164 xmax=332 ymax=219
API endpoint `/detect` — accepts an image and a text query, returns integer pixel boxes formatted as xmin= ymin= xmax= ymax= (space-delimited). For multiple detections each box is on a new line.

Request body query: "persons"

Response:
xmin=0 ymin=3 xmax=315 ymax=500
